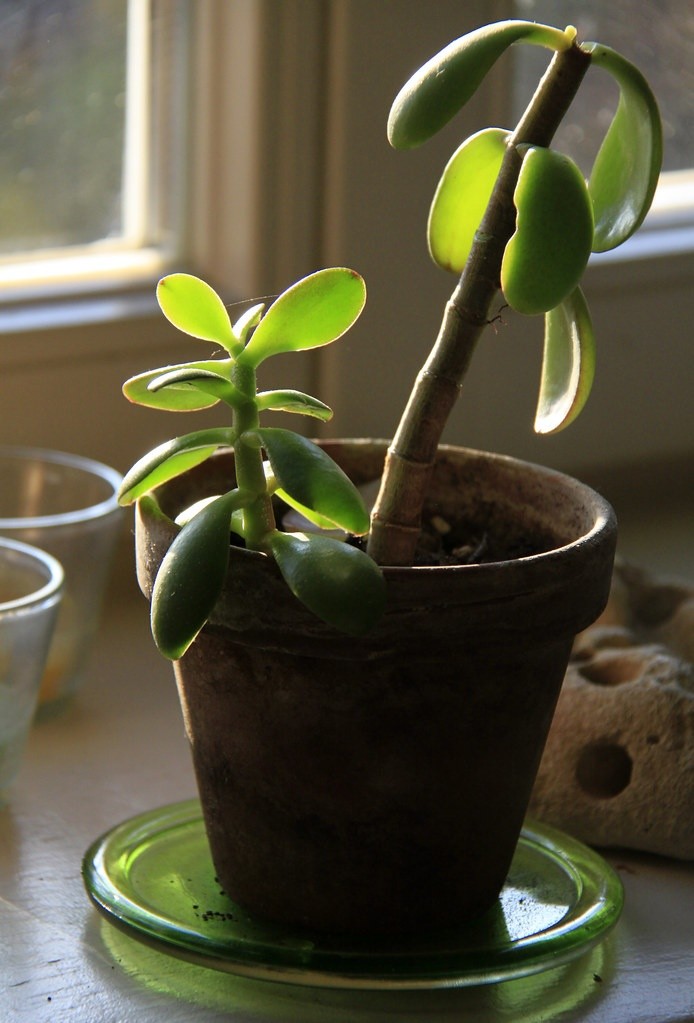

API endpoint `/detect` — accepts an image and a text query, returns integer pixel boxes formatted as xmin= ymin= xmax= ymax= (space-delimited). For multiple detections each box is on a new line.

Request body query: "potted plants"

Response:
xmin=115 ymin=21 xmax=663 ymax=941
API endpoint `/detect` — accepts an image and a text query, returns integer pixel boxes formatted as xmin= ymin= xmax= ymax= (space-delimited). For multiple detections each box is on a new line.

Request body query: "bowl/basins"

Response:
xmin=1 ymin=443 xmax=127 ymax=707
xmin=0 ymin=538 xmax=64 ymax=797
